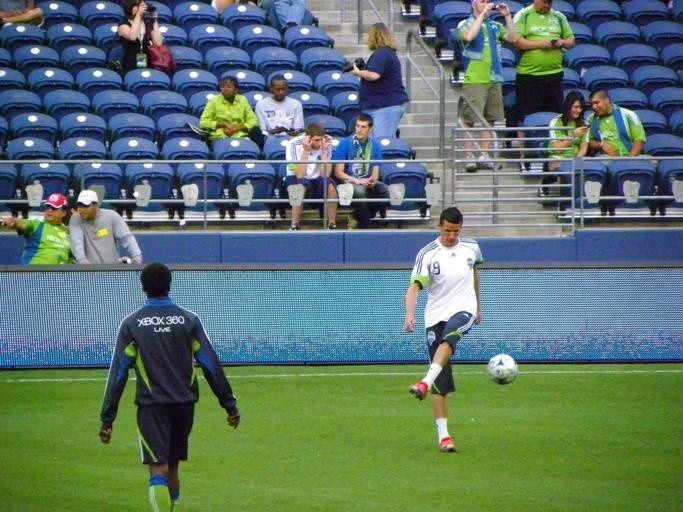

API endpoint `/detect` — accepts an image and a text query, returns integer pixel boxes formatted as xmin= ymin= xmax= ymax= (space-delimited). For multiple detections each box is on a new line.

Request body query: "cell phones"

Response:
xmin=584 ymin=120 xmax=590 ymax=128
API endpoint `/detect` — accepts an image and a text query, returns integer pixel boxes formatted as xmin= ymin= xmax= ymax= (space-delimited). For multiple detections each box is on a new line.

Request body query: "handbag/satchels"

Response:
xmin=148 ymin=45 xmax=176 ymax=71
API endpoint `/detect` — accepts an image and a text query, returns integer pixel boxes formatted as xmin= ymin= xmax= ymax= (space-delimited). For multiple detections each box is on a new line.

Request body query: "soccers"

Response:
xmin=488 ymin=354 xmax=518 ymax=384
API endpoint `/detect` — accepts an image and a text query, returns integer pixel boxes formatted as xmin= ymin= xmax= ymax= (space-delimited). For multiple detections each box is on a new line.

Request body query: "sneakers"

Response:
xmin=464 ymin=155 xmax=477 ymax=172
xmin=439 ymin=436 xmax=455 ymax=449
xmin=478 ymin=152 xmax=503 ymax=170
xmin=408 ymin=380 xmax=428 ymax=401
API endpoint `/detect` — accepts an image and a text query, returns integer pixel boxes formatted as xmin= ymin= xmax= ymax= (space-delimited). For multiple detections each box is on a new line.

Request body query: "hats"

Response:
xmin=76 ymin=190 xmax=98 ymax=205
xmin=44 ymin=192 xmax=68 ymax=209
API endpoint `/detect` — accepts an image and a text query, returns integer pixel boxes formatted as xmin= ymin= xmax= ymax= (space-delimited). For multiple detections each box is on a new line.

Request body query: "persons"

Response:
xmin=511 ymin=0 xmax=575 ymax=174
xmin=207 ymin=1 xmax=258 ymax=15
xmin=457 ymin=1 xmax=518 ymax=172
xmin=334 ymin=113 xmax=390 ymax=229
xmin=99 ymin=263 xmax=240 ymax=512
xmin=403 ymin=208 xmax=485 ymax=450
xmin=201 ymin=76 xmax=259 ymax=140
xmin=285 ymin=123 xmax=339 ymax=231
xmin=0 ymin=192 xmax=75 ymax=264
xmin=0 ymin=0 xmax=46 ymax=30
xmin=350 ymin=24 xmax=409 ymax=137
xmin=586 ymin=89 xmax=659 ymax=168
xmin=538 ymin=91 xmax=591 ymax=216
xmin=254 ymin=74 xmax=305 ymax=146
xmin=69 ymin=189 xmax=142 ymax=263
xmin=118 ymin=0 xmax=164 ymax=77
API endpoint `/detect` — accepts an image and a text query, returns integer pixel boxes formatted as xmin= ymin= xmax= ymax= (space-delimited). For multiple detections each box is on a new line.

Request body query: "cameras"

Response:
xmin=145 ymin=3 xmax=156 ymax=13
xmin=341 ymin=58 xmax=365 ymax=73
xmin=492 ymin=4 xmax=498 ymax=10
